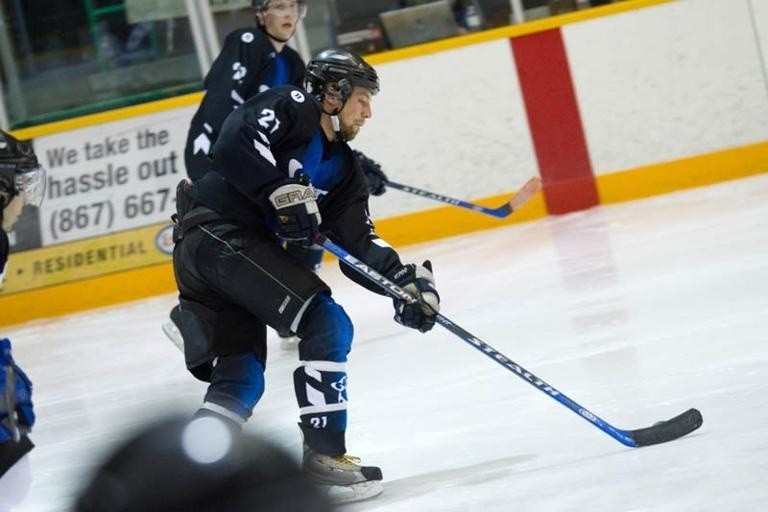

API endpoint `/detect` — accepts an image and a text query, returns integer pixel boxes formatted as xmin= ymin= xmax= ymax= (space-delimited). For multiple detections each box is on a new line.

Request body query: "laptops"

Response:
xmin=378 ymin=1 xmax=459 ymax=49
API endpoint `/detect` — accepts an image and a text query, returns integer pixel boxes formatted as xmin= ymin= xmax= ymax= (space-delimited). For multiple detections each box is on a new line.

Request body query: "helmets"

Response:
xmin=1 ymin=128 xmax=43 ymax=210
xmin=252 ymin=1 xmax=308 ymax=18
xmin=302 ymin=47 xmax=380 ymax=102
xmin=66 ymin=407 xmax=343 ymax=511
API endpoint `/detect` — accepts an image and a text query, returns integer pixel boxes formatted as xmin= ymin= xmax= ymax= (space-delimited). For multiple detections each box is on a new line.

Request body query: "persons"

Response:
xmin=174 ymin=47 xmax=439 ymax=485
xmin=0 ymin=127 xmax=46 ymax=480
xmin=171 ymin=0 xmax=386 ymax=382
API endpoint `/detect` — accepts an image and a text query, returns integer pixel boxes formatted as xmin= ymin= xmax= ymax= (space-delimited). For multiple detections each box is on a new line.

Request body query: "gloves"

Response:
xmin=268 ymin=168 xmax=330 ymax=248
xmin=352 ymin=149 xmax=390 ymax=196
xmin=385 ymin=259 xmax=440 ymax=334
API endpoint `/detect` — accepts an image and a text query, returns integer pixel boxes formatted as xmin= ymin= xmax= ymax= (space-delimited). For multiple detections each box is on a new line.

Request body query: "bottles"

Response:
xmin=366 ymin=21 xmax=385 ymax=52
xmin=466 ymin=5 xmax=481 ymax=32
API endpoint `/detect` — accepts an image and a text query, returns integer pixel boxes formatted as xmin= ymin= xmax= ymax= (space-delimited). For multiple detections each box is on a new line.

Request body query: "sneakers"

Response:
xmin=299 ymin=441 xmax=383 ymax=488
